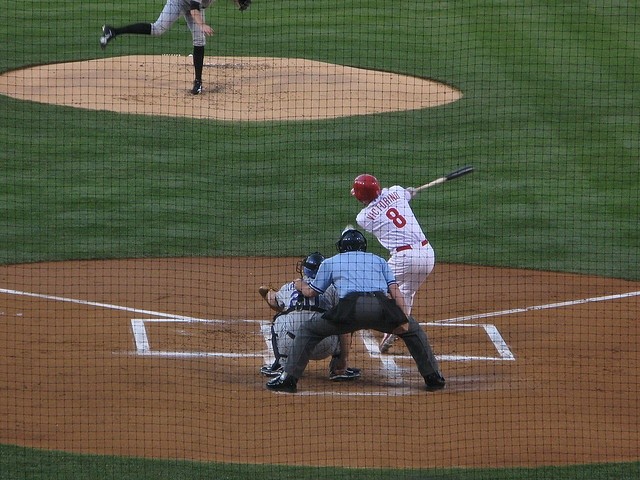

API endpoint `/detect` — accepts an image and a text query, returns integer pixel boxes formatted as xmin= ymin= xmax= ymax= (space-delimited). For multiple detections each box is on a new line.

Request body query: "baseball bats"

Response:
xmin=415 ymin=166 xmax=475 ymax=193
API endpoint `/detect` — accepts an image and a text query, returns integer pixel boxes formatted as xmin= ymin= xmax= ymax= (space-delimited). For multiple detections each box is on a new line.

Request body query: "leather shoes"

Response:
xmin=424 ymin=376 xmax=446 ymax=392
xmin=266 ymin=376 xmax=297 ymax=393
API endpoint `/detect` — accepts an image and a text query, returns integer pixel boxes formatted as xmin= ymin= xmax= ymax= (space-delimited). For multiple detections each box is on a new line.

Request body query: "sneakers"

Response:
xmin=99 ymin=24 xmax=112 ymax=49
xmin=328 ymin=366 xmax=363 ymax=380
xmin=260 ymin=362 xmax=287 ymax=376
xmin=379 ymin=332 xmax=398 ymax=352
xmin=191 ymin=80 xmax=203 ymax=94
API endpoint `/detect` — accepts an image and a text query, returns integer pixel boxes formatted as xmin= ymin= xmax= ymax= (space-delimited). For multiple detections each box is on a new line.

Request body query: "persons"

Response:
xmin=348 ymin=173 xmax=436 ymax=354
xmin=266 ymin=229 xmax=447 ymax=393
xmin=260 ymin=254 xmax=363 ymax=381
xmin=100 ymin=0 xmax=251 ymax=95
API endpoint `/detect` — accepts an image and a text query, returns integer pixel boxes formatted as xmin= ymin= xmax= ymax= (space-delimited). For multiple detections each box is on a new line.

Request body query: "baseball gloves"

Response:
xmin=258 ymin=282 xmax=285 ymax=313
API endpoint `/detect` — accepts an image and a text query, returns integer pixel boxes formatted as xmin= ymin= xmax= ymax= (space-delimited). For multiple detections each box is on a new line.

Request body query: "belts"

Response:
xmin=285 ymin=330 xmax=295 ymax=341
xmin=397 ymin=240 xmax=428 ymax=252
xmin=348 ymin=291 xmax=385 ymax=297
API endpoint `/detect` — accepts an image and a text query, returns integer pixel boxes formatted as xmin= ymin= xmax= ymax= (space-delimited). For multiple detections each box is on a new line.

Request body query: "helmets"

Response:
xmin=350 ymin=174 xmax=381 ymax=201
xmin=296 ymin=252 xmax=324 ymax=279
xmin=336 ymin=230 xmax=367 ymax=252
